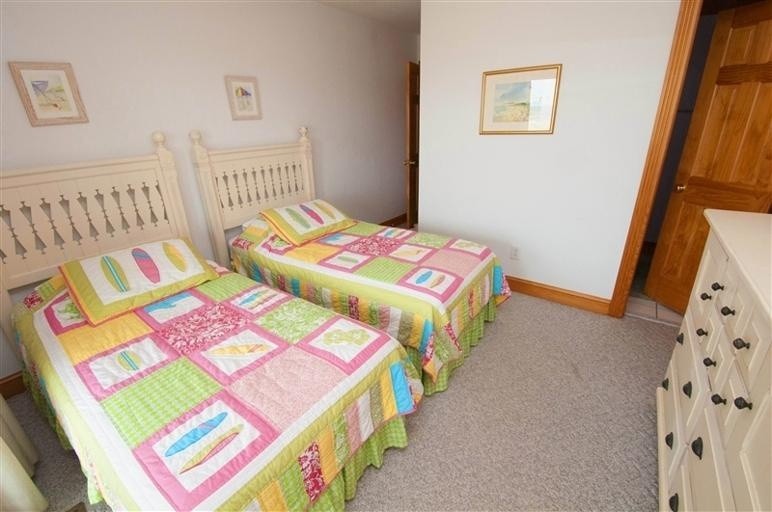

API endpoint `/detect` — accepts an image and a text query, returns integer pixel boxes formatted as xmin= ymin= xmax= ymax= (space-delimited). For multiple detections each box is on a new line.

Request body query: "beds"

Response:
xmin=225 ymin=209 xmax=512 ymax=397
xmin=9 ymin=258 xmax=428 ymax=512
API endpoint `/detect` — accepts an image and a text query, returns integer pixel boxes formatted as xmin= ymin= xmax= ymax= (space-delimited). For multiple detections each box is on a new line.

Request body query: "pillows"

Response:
xmin=57 ymin=237 xmax=220 ymax=328
xmin=257 ymin=198 xmax=356 ymax=247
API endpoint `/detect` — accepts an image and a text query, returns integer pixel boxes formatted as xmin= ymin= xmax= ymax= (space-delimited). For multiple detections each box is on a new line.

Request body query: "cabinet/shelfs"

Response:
xmin=655 ymin=203 xmax=772 ymax=511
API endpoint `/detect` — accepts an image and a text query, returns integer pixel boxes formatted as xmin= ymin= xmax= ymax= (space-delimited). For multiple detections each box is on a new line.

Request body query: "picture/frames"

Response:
xmin=8 ymin=61 xmax=90 ymax=127
xmin=478 ymin=62 xmax=561 ymax=135
xmin=224 ymin=74 xmax=264 ymax=122
